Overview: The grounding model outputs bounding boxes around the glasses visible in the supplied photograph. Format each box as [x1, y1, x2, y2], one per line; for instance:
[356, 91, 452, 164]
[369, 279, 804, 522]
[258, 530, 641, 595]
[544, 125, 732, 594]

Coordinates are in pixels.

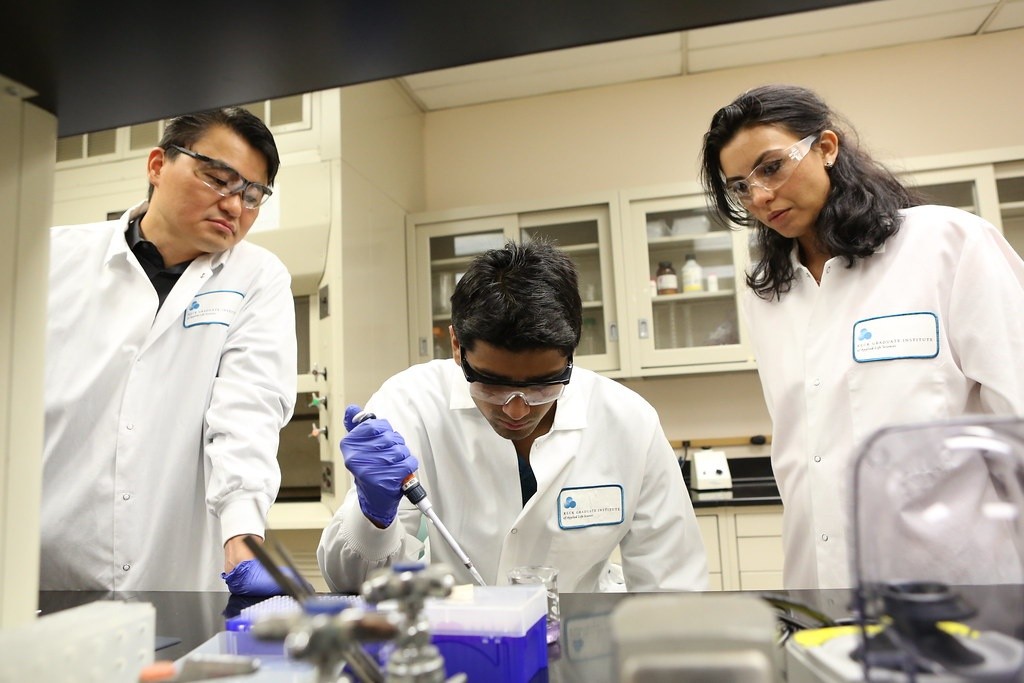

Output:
[726, 131, 821, 210]
[451, 324, 573, 407]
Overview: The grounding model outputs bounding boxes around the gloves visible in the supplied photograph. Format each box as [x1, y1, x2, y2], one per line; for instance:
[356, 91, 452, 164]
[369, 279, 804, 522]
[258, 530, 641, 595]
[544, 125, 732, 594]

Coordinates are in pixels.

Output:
[220, 555, 316, 598]
[164, 142, 273, 210]
[339, 404, 419, 527]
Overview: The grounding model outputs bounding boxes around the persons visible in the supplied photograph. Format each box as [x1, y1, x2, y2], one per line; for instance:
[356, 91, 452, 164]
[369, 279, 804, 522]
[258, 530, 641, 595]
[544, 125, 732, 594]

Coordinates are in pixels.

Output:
[316, 239, 712, 594]
[38, 107, 315, 595]
[700, 84, 1024, 587]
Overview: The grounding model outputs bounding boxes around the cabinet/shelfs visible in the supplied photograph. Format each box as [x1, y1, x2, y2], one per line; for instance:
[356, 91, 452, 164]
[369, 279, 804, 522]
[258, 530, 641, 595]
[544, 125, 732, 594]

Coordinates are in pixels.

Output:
[406, 190, 631, 379]
[620, 148, 1023, 377]
[690, 504, 786, 591]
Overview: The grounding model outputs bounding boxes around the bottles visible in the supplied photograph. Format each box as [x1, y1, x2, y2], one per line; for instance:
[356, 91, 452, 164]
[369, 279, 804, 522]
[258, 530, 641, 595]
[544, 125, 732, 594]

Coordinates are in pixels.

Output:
[681, 254, 704, 294]
[657, 261, 678, 295]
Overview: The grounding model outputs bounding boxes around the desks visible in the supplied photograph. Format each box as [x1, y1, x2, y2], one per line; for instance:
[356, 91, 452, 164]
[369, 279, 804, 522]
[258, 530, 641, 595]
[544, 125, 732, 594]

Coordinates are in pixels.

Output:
[36, 585, 1024, 683]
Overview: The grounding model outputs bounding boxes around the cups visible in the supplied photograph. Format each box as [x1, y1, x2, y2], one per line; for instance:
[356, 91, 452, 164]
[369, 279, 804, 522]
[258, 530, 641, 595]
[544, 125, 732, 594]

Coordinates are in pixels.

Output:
[506, 567, 561, 645]
